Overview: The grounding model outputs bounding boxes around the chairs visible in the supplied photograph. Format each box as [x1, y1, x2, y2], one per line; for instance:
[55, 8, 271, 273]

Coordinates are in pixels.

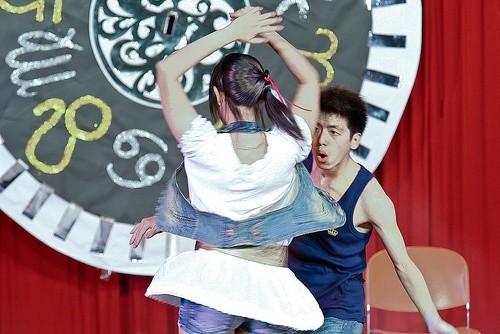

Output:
[366, 246, 481, 334]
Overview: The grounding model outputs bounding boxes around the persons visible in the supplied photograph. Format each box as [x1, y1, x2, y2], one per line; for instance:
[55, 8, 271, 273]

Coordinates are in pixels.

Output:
[144, 8, 346, 334]
[128, 87, 463, 334]
